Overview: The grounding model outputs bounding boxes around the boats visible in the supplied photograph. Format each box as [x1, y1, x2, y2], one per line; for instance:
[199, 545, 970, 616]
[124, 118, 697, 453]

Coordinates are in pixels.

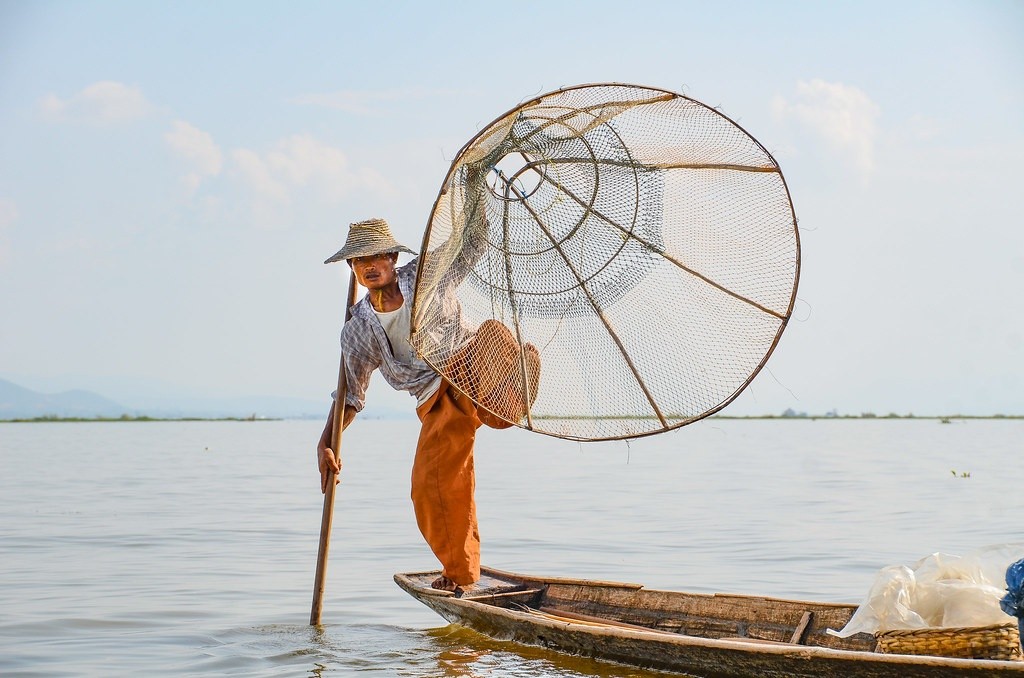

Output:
[391, 563, 1024, 678]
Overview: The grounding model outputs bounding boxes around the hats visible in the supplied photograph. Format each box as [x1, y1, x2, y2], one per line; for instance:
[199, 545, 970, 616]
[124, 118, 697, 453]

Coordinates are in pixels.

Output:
[324, 219, 419, 264]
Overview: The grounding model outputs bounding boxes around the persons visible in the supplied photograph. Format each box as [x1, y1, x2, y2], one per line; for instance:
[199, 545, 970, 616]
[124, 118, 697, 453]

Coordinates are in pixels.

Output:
[318, 161, 539, 589]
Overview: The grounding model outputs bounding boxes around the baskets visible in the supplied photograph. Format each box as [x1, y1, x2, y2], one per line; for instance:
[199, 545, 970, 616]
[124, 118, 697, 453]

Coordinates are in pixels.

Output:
[874, 622, 1022, 663]
